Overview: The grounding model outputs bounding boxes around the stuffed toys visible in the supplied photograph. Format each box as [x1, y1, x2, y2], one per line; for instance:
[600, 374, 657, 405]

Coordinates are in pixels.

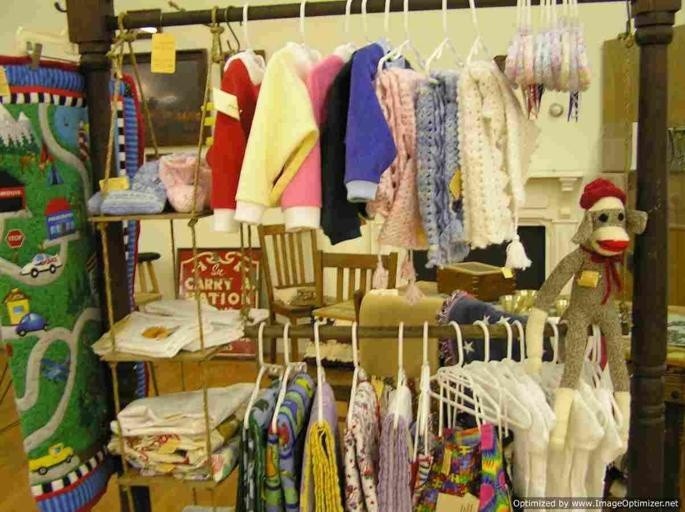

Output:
[524, 178, 648, 450]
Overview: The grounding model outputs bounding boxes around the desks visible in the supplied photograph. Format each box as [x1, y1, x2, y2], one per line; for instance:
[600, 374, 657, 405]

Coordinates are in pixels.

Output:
[311, 280, 684, 510]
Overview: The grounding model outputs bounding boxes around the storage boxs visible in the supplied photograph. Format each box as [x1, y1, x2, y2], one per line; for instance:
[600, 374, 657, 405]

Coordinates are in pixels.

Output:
[436, 260, 516, 302]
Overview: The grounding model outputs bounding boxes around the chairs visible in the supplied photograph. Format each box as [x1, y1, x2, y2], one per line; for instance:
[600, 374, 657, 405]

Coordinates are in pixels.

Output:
[302, 250, 398, 421]
[136, 251, 162, 305]
[257, 223, 336, 364]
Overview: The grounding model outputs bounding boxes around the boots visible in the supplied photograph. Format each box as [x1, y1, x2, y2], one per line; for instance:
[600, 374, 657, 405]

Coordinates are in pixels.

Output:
[159, 153, 204, 214]
